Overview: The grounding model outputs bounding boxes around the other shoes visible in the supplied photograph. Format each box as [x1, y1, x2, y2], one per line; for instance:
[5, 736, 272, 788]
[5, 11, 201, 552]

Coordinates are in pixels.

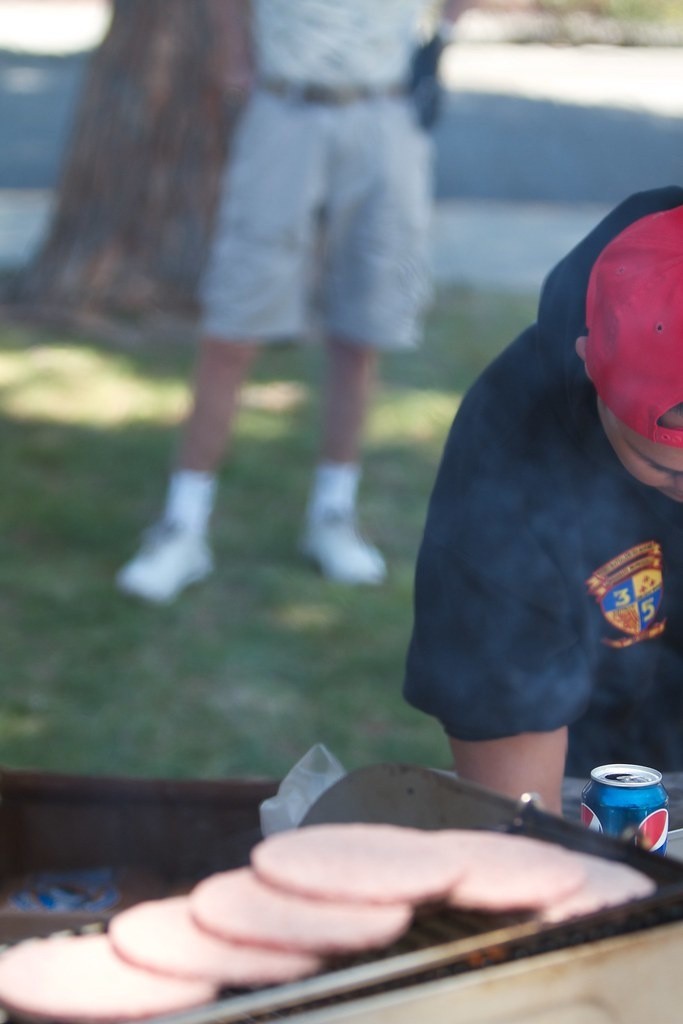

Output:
[114, 518, 214, 604]
[297, 508, 385, 585]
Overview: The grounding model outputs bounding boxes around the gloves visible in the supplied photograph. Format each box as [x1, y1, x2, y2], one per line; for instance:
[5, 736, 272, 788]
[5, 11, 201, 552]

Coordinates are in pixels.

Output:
[411, 39, 443, 128]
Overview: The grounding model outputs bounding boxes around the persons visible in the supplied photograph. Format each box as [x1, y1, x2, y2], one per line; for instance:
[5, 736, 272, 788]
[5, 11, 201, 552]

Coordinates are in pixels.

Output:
[403, 182, 682, 823]
[116, 0, 478, 610]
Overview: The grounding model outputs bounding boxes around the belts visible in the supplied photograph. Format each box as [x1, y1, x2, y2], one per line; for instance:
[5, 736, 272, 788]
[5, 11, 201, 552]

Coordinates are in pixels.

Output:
[255, 78, 411, 107]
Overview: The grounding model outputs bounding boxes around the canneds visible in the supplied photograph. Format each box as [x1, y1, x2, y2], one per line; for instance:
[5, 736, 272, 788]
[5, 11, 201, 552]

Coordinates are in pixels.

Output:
[580, 764, 669, 858]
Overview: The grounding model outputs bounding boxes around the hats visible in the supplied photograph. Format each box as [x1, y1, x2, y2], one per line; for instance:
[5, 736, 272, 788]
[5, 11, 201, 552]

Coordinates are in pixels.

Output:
[586, 204, 683, 448]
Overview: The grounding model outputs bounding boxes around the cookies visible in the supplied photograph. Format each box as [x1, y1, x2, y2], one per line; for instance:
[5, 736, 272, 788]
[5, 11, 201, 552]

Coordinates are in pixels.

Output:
[1, 820, 657, 1024]
